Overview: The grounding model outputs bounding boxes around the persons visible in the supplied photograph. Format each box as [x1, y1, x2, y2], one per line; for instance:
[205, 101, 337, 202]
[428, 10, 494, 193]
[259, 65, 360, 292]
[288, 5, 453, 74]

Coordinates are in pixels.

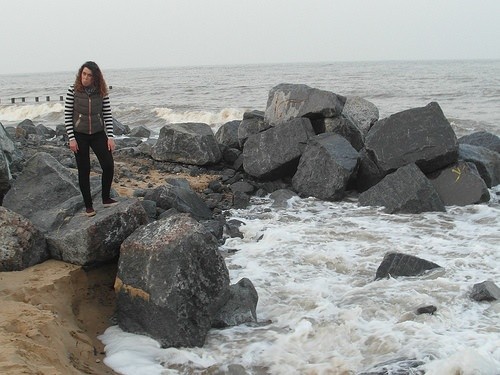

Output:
[63, 60, 121, 218]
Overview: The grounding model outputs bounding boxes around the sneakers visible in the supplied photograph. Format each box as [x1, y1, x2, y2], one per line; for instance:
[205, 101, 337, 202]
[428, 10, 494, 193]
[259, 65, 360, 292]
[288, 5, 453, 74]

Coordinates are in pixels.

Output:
[101, 198, 119, 208]
[84, 207, 96, 216]
